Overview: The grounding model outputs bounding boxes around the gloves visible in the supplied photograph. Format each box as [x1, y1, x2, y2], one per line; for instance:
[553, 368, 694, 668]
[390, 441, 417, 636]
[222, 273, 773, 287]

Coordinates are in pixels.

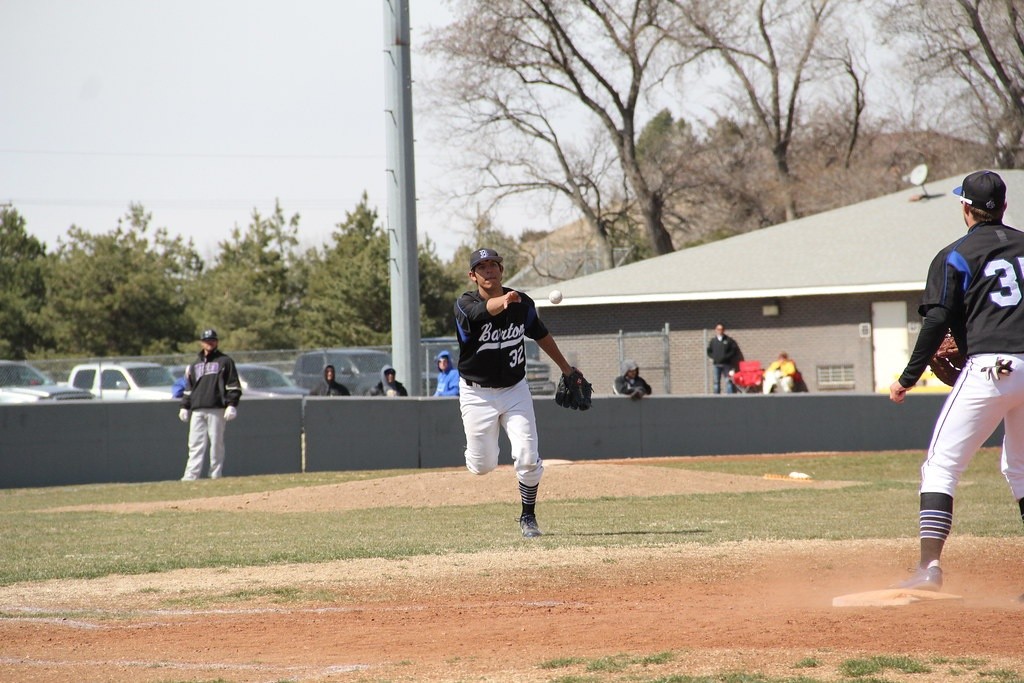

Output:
[178, 408, 189, 422]
[980, 357, 1014, 381]
[223, 406, 237, 421]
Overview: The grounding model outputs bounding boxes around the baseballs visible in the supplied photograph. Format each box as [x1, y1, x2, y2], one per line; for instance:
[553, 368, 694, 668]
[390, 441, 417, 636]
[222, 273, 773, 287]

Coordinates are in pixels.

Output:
[549, 290, 562, 304]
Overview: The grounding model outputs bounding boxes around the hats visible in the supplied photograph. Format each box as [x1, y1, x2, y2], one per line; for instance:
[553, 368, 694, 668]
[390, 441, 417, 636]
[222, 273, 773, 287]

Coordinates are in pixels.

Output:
[952, 169, 1006, 213]
[470, 248, 503, 270]
[201, 329, 218, 341]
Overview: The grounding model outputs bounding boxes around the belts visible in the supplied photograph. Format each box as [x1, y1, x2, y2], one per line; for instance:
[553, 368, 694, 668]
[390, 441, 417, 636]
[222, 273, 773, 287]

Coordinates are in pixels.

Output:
[465, 379, 501, 390]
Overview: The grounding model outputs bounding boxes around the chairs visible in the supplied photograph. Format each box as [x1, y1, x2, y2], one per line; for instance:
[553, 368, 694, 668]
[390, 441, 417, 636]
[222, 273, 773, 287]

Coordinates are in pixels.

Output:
[733, 362, 764, 397]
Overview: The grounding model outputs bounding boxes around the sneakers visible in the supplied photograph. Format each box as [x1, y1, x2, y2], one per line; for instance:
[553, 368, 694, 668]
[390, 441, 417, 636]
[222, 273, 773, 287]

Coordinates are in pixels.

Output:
[894, 564, 944, 591]
[515, 514, 542, 537]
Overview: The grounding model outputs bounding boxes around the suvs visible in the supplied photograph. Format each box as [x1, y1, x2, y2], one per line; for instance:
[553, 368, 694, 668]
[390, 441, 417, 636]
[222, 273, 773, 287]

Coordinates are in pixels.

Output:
[0, 361, 94, 403]
[168, 349, 556, 398]
[66, 361, 192, 400]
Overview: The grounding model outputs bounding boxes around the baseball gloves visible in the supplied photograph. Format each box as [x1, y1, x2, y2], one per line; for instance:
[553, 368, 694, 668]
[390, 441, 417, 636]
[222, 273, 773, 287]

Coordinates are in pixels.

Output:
[928, 327, 965, 387]
[555, 367, 592, 411]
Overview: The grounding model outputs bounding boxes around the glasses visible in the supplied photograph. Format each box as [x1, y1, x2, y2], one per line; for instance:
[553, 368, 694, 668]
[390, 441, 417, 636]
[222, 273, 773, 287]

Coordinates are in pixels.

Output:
[384, 370, 395, 375]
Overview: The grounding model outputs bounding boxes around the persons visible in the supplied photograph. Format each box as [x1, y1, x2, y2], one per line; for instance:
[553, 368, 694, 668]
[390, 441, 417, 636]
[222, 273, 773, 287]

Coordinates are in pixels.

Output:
[454, 248, 593, 537]
[707, 324, 737, 394]
[890, 169, 1024, 590]
[763, 350, 796, 395]
[363, 363, 408, 396]
[178, 328, 243, 482]
[172, 364, 190, 398]
[308, 363, 351, 397]
[432, 349, 459, 397]
[613, 360, 652, 402]
